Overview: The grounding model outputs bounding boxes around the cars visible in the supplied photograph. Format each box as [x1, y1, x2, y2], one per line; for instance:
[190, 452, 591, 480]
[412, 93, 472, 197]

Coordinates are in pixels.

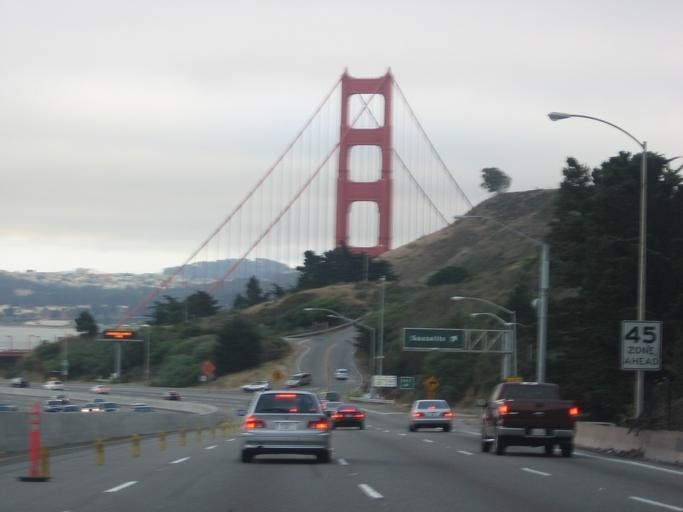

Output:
[238, 389, 365, 463]
[0, 377, 183, 414]
[408, 400, 455, 431]
[333, 367, 350, 382]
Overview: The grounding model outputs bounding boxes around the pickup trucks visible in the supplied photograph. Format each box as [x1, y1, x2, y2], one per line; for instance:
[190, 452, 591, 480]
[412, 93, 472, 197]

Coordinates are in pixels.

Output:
[480, 381, 578, 456]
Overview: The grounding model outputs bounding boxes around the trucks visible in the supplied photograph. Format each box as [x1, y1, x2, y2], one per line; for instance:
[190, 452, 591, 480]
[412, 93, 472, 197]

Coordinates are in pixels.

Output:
[240, 373, 313, 392]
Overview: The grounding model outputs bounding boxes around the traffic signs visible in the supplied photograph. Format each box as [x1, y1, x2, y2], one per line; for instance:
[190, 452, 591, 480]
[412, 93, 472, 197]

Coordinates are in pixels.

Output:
[422, 376, 440, 392]
[404, 326, 515, 355]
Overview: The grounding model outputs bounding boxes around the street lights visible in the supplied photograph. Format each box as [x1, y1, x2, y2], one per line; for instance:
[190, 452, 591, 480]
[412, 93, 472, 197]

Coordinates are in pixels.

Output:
[446, 211, 560, 384]
[549, 110, 655, 419]
[305, 275, 389, 376]
